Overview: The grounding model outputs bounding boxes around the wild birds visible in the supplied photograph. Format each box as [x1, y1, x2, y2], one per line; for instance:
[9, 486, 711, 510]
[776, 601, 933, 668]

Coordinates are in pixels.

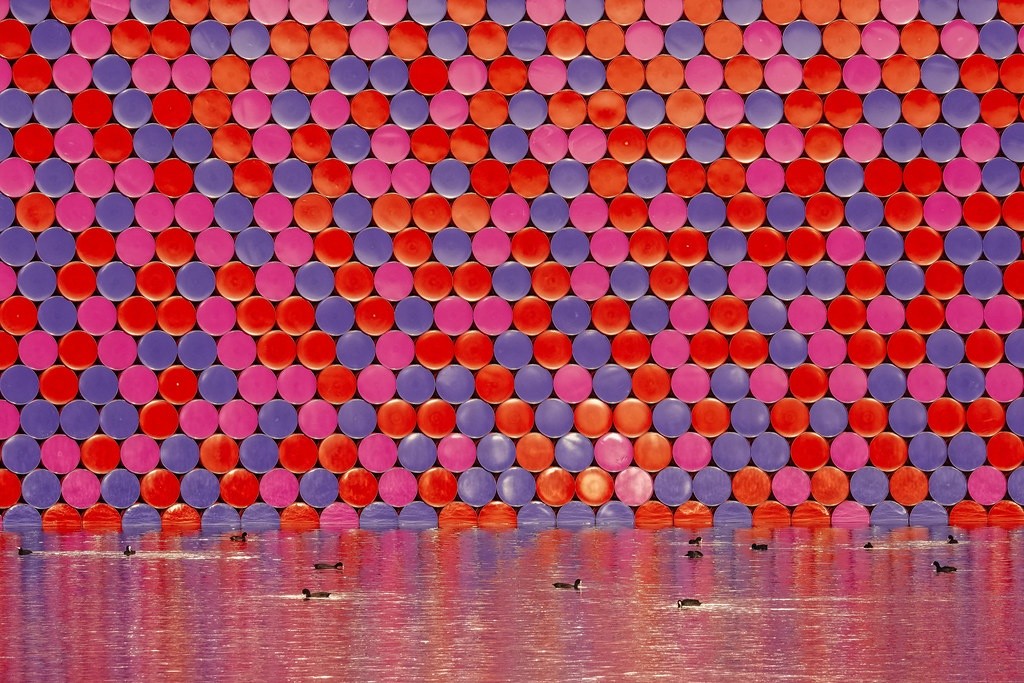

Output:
[124, 545, 136, 556]
[17, 546, 33, 555]
[930, 561, 957, 572]
[312, 562, 345, 569]
[947, 535, 959, 544]
[688, 537, 702, 544]
[230, 532, 248, 541]
[678, 598, 703, 608]
[684, 550, 704, 559]
[302, 589, 331, 598]
[551, 578, 582, 589]
[864, 542, 873, 548]
[752, 543, 768, 551]
